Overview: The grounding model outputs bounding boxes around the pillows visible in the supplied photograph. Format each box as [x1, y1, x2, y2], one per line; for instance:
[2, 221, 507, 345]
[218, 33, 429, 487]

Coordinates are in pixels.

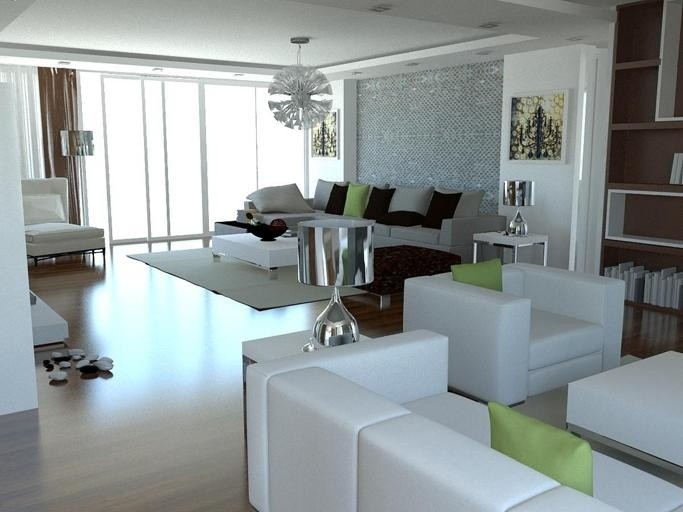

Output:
[451, 258, 503, 291]
[486, 400, 593, 498]
[245, 179, 483, 229]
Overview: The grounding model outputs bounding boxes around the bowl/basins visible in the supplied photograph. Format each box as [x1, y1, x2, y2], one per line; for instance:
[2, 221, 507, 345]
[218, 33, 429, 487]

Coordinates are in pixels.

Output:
[246, 223, 287, 240]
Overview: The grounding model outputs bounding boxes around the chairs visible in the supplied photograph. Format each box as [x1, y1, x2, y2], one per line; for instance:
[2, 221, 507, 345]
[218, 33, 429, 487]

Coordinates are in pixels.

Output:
[402, 262, 624, 406]
[20, 177, 107, 270]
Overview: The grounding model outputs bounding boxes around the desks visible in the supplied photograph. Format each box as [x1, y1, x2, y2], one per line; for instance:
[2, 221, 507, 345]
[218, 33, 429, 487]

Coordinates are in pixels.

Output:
[471, 231, 549, 267]
[242, 329, 373, 388]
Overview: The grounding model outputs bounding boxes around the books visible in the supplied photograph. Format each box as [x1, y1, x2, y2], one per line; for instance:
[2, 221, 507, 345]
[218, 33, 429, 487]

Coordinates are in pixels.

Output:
[668, 152, 683, 184]
[621, 306, 680, 350]
[601, 258, 683, 310]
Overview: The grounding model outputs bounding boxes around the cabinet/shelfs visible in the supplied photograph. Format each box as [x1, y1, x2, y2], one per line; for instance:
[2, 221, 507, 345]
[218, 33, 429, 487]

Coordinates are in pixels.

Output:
[602, 0, 681, 315]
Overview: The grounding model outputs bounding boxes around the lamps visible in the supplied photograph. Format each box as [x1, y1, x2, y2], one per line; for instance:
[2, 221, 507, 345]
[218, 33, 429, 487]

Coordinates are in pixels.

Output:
[503, 178, 535, 237]
[59, 128, 94, 225]
[266, 38, 332, 132]
[295, 221, 376, 351]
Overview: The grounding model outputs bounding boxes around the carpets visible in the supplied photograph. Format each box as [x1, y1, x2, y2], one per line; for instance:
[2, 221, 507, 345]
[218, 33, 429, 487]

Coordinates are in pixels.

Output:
[125, 247, 367, 311]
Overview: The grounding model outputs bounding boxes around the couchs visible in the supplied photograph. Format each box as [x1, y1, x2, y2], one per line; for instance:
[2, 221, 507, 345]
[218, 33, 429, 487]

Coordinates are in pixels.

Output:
[244, 328, 681, 512]
[215, 198, 498, 265]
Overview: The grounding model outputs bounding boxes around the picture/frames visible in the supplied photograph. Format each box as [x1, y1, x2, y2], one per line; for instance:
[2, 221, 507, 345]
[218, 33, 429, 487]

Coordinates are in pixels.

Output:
[309, 109, 339, 162]
[505, 89, 569, 163]
